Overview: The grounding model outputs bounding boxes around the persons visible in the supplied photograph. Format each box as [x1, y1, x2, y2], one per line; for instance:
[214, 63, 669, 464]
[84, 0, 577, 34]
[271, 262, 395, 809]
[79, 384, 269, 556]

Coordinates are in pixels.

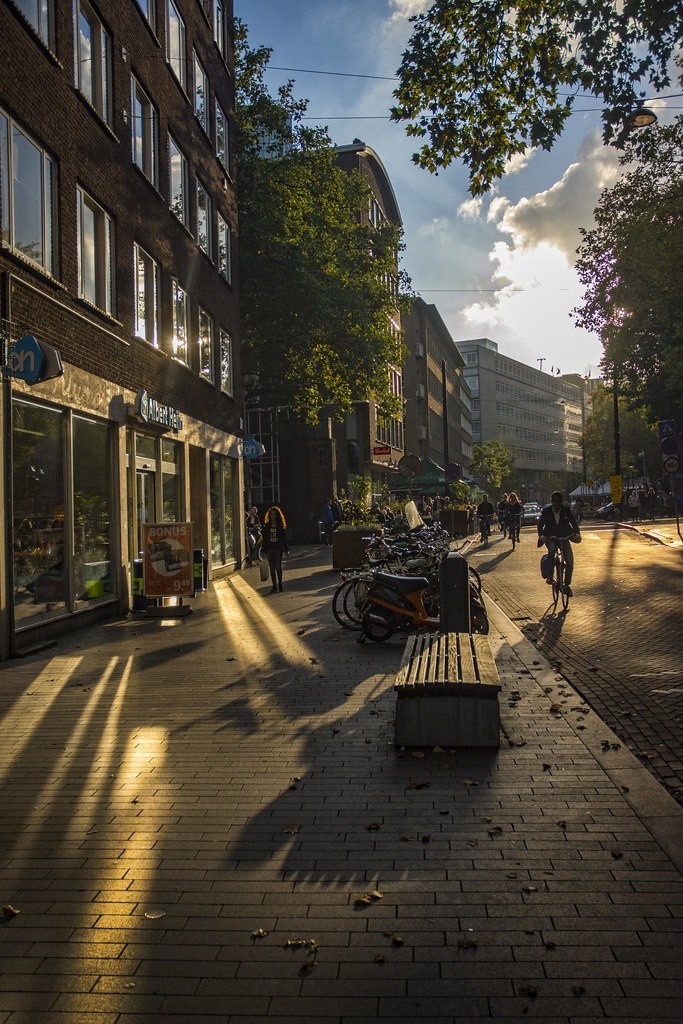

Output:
[262, 506, 290, 593]
[14, 519, 64, 578]
[320, 493, 457, 548]
[477, 495, 494, 542]
[573, 497, 584, 522]
[244, 507, 261, 567]
[537, 491, 582, 597]
[628, 488, 675, 524]
[496, 494, 509, 532]
[506, 492, 522, 543]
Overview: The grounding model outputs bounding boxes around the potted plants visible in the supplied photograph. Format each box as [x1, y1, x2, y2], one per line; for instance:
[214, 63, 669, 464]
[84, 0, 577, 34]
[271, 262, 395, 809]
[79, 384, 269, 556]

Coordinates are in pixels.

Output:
[434, 503, 469, 539]
[332, 522, 383, 572]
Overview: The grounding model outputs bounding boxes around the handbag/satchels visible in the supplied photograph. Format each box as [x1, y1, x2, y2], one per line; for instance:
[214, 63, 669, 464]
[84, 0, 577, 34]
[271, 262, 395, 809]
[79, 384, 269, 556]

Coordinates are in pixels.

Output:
[260, 554, 269, 582]
[541, 554, 551, 579]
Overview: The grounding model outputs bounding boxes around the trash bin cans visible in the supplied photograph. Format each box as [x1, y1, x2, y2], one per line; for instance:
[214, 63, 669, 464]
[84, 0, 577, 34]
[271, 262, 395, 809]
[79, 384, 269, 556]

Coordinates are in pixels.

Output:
[439, 552, 472, 634]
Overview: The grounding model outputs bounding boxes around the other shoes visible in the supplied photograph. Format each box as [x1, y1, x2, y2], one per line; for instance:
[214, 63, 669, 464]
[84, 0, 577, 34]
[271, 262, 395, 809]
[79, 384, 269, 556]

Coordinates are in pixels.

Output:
[546, 577, 553, 584]
[278, 582, 283, 592]
[271, 587, 277, 594]
[560, 586, 572, 597]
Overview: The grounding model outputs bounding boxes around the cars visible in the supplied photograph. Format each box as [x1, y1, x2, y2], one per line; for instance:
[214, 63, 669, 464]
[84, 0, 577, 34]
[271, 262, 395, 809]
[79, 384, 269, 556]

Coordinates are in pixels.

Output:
[521, 502, 543, 526]
[597, 501, 639, 520]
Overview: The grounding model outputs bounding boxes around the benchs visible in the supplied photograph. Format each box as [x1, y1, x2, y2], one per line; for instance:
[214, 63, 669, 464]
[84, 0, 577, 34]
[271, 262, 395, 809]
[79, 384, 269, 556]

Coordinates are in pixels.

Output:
[393, 631, 504, 753]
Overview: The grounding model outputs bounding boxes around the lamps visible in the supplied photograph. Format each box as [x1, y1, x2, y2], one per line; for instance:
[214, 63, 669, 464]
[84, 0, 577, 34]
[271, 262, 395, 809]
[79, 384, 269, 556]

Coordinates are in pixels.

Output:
[626, 100, 657, 128]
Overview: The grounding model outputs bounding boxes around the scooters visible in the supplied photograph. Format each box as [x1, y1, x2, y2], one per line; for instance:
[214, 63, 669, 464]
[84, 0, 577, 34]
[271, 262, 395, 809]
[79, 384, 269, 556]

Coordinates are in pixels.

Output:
[360, 569, 489, 641]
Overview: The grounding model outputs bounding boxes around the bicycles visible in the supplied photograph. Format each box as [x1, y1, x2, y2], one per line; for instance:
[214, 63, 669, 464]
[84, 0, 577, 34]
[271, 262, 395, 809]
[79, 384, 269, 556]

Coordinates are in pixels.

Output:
[332, 521, 489, 642]
[245, 525, 270, 565]
[480, 512, 522, 551]
[545, 535, 576, 609]
